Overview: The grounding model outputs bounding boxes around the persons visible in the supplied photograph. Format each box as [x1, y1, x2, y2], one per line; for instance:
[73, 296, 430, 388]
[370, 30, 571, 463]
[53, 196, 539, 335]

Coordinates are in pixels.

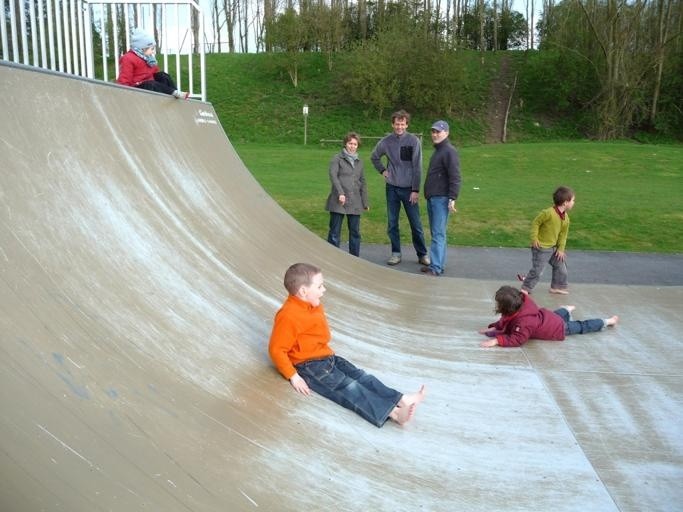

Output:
[424, 119, 462, 276]
[268, 263, 426, 429]
[322, 132, 370, 259]
[371, 109, 431, 268]
[114, 26, 191, 99]
[478, 286, 618, 346]
[521, 187, 577, 296]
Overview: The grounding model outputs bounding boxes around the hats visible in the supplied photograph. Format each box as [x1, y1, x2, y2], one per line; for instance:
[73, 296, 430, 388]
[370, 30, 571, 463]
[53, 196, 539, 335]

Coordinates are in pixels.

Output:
[431, 120, 449, 133]
[130, 27, 156, 50]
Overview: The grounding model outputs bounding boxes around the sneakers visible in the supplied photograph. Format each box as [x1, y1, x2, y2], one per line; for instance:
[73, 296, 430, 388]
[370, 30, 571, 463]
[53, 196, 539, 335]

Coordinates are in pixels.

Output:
[387, 255, 401, 265]
[418, 254, 430, 265]
